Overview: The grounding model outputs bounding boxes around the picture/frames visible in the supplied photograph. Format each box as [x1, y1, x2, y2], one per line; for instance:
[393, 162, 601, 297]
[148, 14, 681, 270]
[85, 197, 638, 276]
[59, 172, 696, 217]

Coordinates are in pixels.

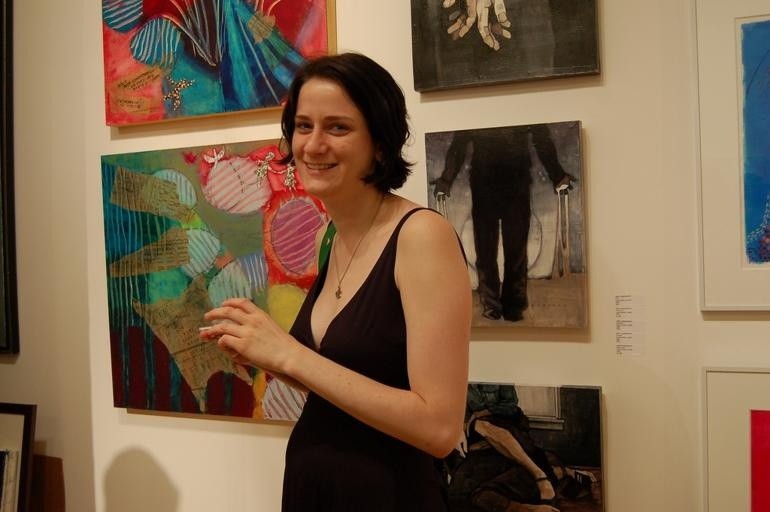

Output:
[693, 1, 770, 315]
[701, 366, 769, 512]
[0, 402, 38, 511]
[0, 0, 20, 357]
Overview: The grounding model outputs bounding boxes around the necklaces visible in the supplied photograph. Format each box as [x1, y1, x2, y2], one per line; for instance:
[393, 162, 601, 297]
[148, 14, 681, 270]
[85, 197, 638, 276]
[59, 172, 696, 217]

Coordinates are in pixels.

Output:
[333, 193, 386, 299]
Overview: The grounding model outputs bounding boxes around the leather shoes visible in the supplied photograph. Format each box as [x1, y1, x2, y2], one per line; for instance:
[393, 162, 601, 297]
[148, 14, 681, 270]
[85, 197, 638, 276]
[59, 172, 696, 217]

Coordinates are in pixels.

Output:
[481, 307, 523, 321]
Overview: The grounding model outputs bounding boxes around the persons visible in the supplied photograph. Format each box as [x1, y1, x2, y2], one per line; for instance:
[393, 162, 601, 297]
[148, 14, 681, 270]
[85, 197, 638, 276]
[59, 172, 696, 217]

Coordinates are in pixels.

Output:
[200, 54, 474, 512]
[429, 125, 577, 322]
[442, 0, 511, 51]
[455, 385, 558, 506]
